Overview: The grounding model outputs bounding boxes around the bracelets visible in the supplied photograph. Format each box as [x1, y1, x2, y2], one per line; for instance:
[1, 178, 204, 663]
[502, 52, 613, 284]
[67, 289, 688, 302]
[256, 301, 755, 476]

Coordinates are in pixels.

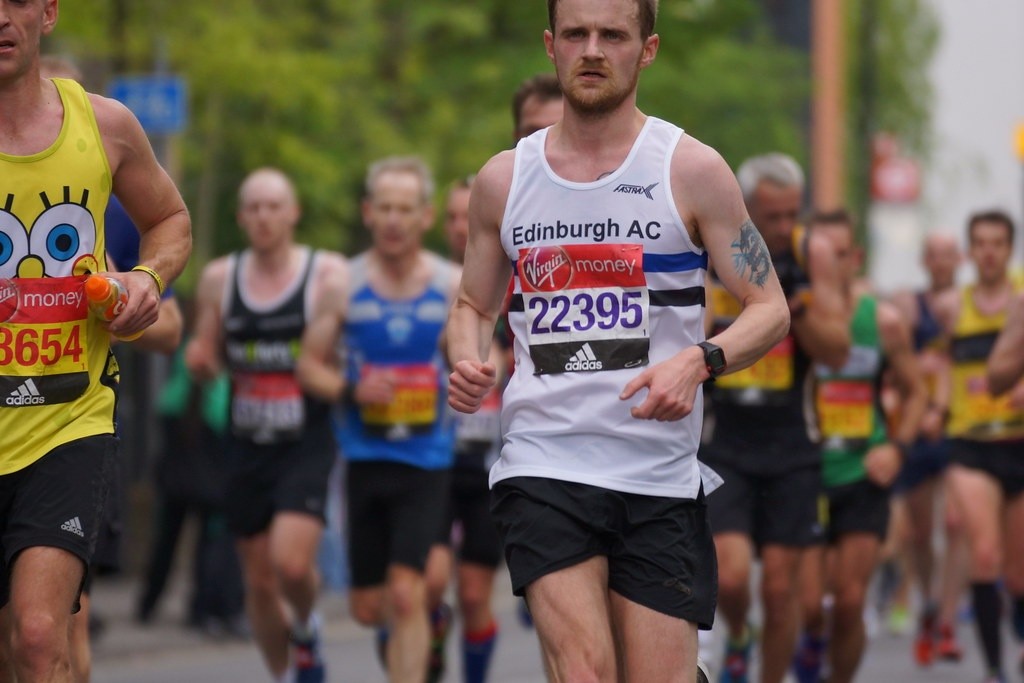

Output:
[341, 377, 357, 404]
[131, 266, 164, 296]
[892, 438, 908, 459]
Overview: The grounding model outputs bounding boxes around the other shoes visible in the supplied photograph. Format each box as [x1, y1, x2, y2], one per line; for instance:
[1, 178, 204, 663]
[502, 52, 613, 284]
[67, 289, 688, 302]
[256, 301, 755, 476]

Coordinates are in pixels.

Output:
[427, 602, 453, 683]
[792, 631, 828, 683]
[882, 604, 909, 637]
[717, 623, 754, 683]
[861, 604, 881, 641]
[694, 657, 711, 683]
[288, 613, 326, 683]
[375, 627, 389, 669]
[910, 620, 963, 669]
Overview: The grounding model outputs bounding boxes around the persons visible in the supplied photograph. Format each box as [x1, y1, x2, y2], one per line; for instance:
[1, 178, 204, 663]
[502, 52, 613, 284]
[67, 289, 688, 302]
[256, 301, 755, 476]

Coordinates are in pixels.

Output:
[293, 154, 462, 682]
[427, 311, 516, 683]
[186, 167, 348, 682]
[89, 197, 184, 636]
[445, 175, 516, 682]
[442, 0, 790, 683]
[512, 73, 564, 146]
[0, 0, 194, 682]
[698, 152, 1024, 683]
[133, 297, 252, 638]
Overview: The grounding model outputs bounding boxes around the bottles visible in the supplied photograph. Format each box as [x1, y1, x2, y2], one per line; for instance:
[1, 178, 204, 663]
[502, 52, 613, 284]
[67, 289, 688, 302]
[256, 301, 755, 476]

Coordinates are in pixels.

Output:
[85, 276, 147, 342]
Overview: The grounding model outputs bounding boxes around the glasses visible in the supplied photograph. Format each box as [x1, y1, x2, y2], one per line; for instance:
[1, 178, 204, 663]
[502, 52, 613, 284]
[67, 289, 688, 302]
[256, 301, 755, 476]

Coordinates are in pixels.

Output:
[836, 250, 850, 259]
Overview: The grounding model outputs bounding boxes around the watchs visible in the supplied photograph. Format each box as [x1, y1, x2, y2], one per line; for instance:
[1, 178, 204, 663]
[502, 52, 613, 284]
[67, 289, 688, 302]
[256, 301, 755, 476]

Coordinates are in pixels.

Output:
[698, 342, 727, 378]
